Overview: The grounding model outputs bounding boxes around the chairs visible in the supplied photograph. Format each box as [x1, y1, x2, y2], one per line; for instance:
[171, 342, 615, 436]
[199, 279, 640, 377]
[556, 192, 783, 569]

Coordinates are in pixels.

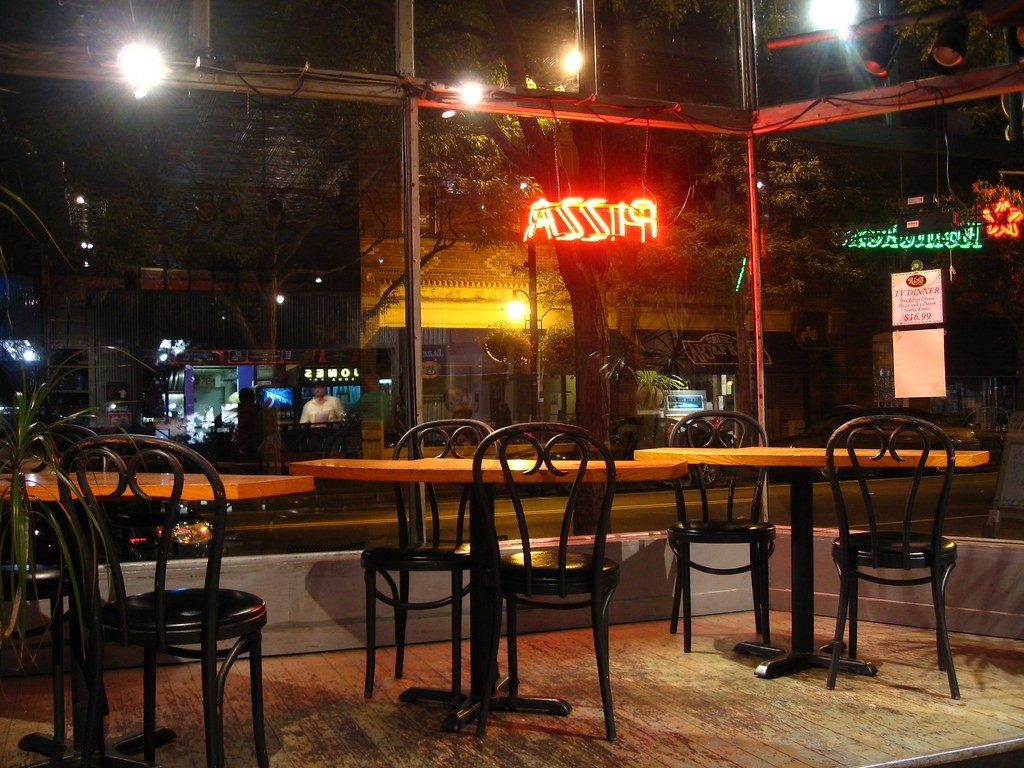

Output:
[0, 422, 111, 754]
[667, 410, 776, 652]
[359, 418, 501, 708]
[57, 433, 270, 768]
[826, 415, 962, 701]
[472, 421, 622, 740]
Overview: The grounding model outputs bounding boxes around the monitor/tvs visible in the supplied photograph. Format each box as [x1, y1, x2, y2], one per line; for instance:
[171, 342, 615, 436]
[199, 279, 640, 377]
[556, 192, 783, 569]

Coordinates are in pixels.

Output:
[256, 385, 299, 409]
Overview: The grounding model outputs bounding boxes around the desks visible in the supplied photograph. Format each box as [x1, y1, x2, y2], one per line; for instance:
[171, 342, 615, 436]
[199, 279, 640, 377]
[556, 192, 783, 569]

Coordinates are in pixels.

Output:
[0, 469, 316, 768]
[632, 448, 989, 680]
[288, 458, 690, 731]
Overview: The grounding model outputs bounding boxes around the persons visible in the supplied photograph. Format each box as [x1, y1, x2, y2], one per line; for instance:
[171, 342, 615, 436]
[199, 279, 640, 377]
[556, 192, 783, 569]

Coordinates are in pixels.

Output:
[299, 386, 346, 427]
